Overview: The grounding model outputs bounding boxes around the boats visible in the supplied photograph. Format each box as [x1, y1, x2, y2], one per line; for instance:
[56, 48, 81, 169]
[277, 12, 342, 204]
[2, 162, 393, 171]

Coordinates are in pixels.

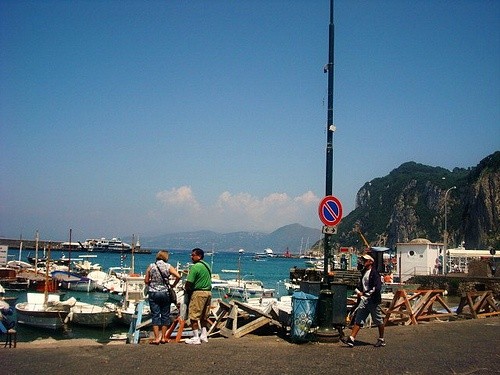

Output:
[184, 289, 421, 315]
[205, 250, 214, 255]
[69, 302, 117, 330]
[84, 237, 130, 249]
[115, 272, 178, 328]
[61, 240, 81, 245]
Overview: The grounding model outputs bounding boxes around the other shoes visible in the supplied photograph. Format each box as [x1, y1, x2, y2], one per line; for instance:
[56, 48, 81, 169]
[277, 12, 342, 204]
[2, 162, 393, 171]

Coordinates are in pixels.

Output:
[185, 337, 201, 345]
[199, 334, 209, 343]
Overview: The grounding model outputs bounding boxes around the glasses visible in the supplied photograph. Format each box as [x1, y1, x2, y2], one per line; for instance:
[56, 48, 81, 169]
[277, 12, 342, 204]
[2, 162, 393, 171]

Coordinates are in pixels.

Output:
[192, 254, 198, 257]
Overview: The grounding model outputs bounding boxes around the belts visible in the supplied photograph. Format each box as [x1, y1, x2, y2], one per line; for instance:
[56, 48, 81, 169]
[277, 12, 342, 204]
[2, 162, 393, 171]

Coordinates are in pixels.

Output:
[193, 288, 211, 291]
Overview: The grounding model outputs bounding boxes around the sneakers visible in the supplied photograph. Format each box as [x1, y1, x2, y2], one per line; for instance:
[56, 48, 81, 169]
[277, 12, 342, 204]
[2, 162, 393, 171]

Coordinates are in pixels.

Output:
[340, 336, 354, 347]
[374, 338, 386, 347]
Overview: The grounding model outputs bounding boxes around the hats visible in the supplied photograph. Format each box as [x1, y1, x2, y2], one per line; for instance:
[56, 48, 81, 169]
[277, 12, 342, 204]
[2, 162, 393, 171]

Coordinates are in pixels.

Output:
[361, 254, 374, 262]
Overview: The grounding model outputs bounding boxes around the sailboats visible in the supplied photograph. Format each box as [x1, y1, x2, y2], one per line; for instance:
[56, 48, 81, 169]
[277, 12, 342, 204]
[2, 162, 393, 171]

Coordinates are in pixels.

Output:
[8, 226, 275, 296]
[14, 244, 70, 332]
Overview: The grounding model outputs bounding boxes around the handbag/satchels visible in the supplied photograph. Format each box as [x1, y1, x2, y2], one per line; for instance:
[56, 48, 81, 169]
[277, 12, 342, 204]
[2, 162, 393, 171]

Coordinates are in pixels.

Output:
[168, 286, 177, 303]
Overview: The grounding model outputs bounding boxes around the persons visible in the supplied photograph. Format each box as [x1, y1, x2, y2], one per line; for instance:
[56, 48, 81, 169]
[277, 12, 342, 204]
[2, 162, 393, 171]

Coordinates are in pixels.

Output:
[185, 248, 212, 345]
[145, 250, 181, 343]
[339, 252, 386, 348]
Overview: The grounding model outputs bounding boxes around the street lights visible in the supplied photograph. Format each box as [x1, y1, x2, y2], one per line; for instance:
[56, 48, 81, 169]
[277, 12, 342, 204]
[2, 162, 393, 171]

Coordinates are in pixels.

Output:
[443, 186, 457, 273]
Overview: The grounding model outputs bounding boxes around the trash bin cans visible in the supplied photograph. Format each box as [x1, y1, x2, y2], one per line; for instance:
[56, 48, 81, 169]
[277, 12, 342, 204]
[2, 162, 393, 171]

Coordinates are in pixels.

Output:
[329, 283, 348, 324]
[291, 292, 318, 342]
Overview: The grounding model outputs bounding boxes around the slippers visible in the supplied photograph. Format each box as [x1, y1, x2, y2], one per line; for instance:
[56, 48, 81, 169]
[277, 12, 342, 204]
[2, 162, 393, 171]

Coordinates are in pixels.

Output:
[160, 339, 165, 344]
[149, 340, 159, 345]
